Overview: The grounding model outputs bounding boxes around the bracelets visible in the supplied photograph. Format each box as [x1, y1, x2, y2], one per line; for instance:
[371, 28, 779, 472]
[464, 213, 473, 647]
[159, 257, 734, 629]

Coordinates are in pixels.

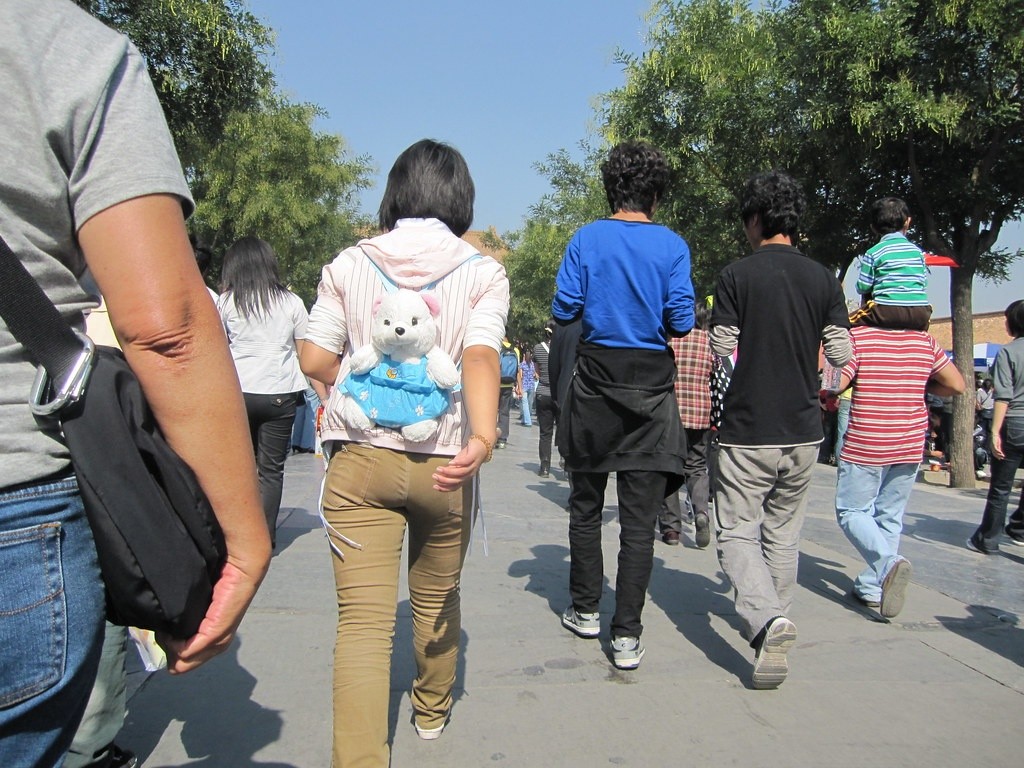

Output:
[991, 434, 1000, 437]
[464, 435, 493, 463]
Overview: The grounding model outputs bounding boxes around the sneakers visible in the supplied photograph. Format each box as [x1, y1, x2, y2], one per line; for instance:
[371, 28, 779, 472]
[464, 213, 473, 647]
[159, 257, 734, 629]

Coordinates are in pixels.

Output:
[966, 537, 998, 554]
[879, 561, 913, 619]
[562, 599, 601, 637]
[612, 637, 646, 669]
[752, 617, 799, 688]
[1005, 529, 1022, 545]
[852, 581, 878, 607]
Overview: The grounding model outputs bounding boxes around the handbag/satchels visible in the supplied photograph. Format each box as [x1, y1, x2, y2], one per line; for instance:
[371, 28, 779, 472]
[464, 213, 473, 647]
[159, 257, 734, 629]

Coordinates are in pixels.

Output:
[30, 336, 227, 642]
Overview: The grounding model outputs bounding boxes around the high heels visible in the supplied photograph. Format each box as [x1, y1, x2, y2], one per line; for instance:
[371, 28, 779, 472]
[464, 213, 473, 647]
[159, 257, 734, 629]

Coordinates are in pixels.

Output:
[292, 446, 313, 454]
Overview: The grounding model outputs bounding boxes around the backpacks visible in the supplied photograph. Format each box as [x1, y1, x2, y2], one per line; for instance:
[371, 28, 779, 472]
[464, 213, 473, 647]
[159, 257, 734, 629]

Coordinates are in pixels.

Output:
[350, 251, 483, 444]
[499, 342, 519, 383]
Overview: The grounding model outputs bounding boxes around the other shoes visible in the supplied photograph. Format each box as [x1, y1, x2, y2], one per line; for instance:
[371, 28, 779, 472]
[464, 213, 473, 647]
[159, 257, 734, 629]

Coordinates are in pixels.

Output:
[695, 512, 711, 548]
[412, 699, 446, 740]
[496, 442, 506, 451]
[662, 531, 682, 544]
[97, 745, 139, 766]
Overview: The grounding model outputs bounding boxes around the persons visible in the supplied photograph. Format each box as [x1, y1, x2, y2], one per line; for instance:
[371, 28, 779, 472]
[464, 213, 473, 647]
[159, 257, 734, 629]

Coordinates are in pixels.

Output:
[1, 1, 1023, 767]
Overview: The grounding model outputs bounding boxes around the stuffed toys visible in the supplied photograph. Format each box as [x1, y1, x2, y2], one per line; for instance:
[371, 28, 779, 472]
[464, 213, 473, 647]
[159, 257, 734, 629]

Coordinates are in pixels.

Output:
[337, 286, 460, 444]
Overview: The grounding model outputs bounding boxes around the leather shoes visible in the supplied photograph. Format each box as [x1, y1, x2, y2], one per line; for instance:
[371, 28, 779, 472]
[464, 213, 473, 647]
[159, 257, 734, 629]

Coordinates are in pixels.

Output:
[538, 469, 550, 478]
